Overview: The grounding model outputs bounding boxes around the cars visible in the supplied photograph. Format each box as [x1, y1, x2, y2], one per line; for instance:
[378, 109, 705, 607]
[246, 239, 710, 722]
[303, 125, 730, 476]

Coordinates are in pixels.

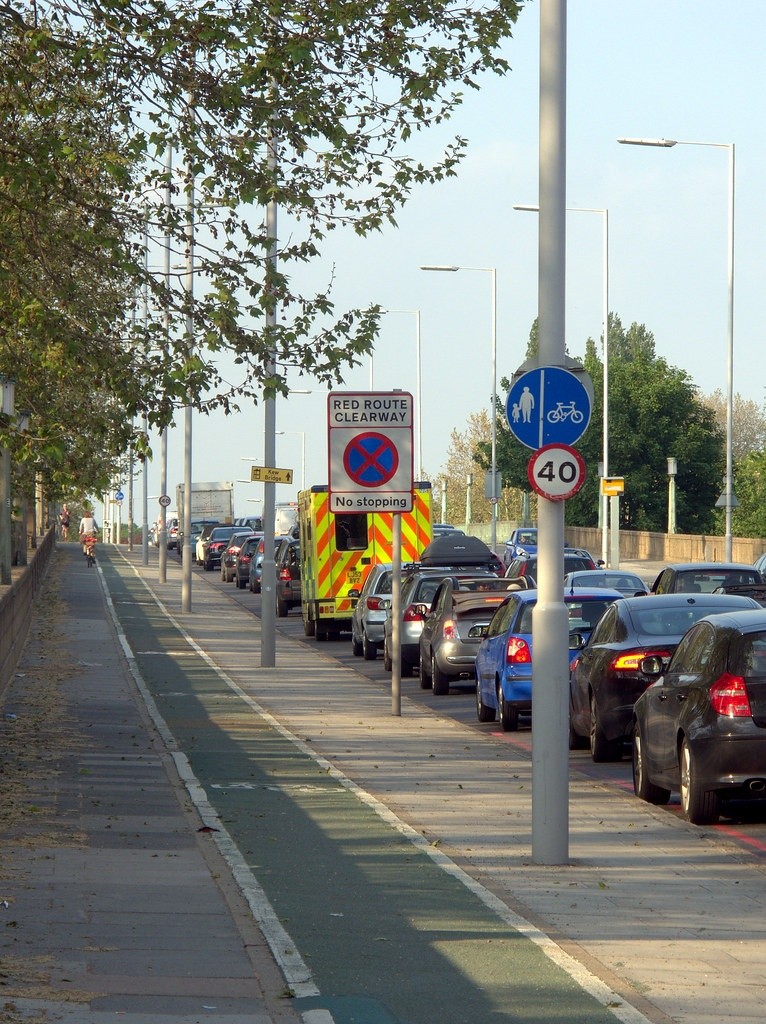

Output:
[564, 571, 652, 629]
[412, 577, 528, 694]
[505, 526, 568, 567]
[632, 564, 765, 608]
[468, 584, 626, 732]
[150, 517, 287, 593]
[712, 584, 766, 609]
[274, 534, 302, 617]
[747, 554, 766, 583]
[568, 592, 764, 764]
[348, 565, 450, 661]
[632, 610, 766, 824]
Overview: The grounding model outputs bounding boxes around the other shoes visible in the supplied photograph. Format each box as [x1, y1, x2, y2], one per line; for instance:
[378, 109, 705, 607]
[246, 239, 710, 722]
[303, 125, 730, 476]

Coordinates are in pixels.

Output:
[62, 536, 64, 541]
[84, 553, 87, 555]
[90, 556, 95, 563]
[65, 537, 67, 543]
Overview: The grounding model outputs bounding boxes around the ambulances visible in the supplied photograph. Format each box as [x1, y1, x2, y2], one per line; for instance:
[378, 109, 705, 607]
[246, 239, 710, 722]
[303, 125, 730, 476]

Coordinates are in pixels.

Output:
[292, 481, 432, 641]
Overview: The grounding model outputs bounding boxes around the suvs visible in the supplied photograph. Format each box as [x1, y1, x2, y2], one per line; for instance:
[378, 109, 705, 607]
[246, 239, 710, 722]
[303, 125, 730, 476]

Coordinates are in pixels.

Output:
[504, 549, 606, 587]
[377, 535, 501, 677]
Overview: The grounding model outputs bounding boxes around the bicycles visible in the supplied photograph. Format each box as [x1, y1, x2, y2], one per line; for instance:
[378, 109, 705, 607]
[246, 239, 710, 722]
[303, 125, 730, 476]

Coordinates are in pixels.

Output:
[87, 545, 94, 568]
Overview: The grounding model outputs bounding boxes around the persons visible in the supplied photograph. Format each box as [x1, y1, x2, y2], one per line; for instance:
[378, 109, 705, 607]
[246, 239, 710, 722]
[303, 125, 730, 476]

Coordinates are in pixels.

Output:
[519, 536, 536, 544]
[79, 511, 99, 564]
[59, 504, 71, 538]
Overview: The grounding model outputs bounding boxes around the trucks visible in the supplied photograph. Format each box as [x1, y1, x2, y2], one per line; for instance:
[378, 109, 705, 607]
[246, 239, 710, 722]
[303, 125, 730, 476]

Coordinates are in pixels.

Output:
[273, 502, 299, 536]
[176, 482, 234, 555]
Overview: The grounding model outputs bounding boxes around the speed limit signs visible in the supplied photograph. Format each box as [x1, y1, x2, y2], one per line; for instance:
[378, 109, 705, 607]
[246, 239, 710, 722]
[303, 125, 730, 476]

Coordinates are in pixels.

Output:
[527, 444, 585, 500]
[117, 500, 123, 506]
[159, 496, 171, 507]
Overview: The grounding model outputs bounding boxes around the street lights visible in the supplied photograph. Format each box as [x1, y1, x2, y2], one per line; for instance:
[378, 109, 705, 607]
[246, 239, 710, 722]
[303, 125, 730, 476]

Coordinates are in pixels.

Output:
[274, 430, 305, 492]
[511, 203, 608, 561]
[420, 264, 497, 551]
[247, 499, 263, 518]
[360, 309, 422, 484]
[620, 135, 735, 563]
